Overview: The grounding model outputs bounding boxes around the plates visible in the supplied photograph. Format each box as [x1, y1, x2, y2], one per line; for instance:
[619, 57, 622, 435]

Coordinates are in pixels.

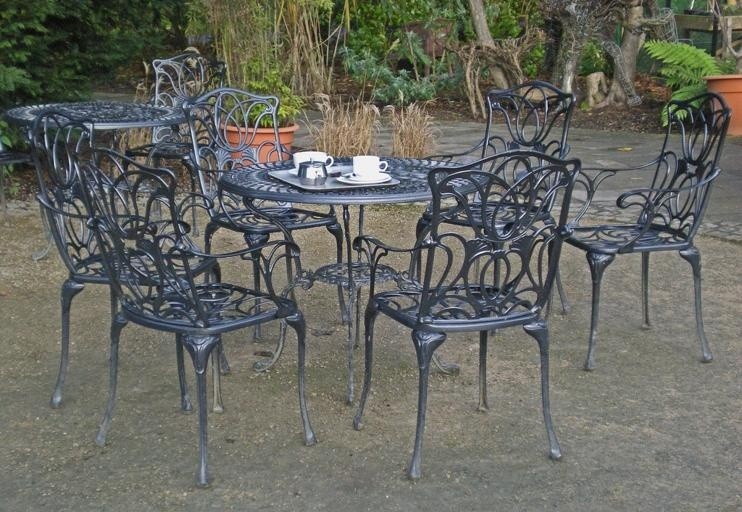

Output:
[288, 167, 339, 177]
[337, 172, 392, 185]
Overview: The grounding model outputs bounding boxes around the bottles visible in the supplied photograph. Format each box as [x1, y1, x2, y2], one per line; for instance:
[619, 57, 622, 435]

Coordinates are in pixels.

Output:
[298, 158, 328, 186]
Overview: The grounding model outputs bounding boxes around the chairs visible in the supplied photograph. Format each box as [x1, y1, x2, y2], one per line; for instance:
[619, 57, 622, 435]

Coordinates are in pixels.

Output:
[351, 148, 582, 481]
[546, 93, 732, 372]
[70, 146, 318, 486]
[182, 87, 352, 344]
[416, 79, 576, 317]
[8, 53, 228, 272]
[22, 106, 230, 413]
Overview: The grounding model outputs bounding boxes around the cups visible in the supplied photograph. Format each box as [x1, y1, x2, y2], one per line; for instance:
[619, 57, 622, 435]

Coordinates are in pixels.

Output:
[352, 155, 388, 179]
[292, 151, 335, 171]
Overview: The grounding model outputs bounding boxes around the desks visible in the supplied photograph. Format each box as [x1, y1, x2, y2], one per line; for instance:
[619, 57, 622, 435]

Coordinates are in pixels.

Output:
[217, 154, 491, 404]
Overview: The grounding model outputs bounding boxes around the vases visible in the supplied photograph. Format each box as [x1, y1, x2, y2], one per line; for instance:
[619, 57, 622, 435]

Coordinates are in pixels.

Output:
[704, 77, 742, 137]
